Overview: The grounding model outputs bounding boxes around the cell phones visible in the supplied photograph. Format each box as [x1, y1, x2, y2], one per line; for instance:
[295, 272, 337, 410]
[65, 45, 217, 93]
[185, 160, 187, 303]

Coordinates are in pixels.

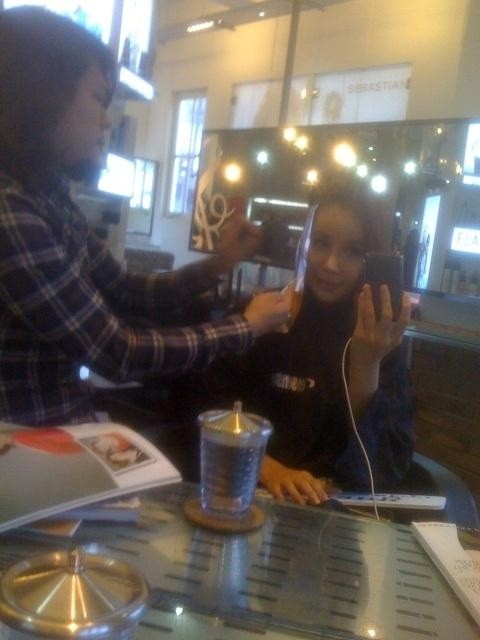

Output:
[365, 253, 403, 322]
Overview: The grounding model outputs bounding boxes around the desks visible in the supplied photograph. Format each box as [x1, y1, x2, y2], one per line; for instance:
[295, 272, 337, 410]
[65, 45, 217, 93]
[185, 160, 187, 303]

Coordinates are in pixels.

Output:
[0, 482, 480, 640]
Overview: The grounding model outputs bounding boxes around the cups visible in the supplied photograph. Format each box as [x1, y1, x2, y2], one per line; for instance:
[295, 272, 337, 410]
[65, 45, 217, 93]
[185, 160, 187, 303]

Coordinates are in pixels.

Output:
[196, 400, 274, 520]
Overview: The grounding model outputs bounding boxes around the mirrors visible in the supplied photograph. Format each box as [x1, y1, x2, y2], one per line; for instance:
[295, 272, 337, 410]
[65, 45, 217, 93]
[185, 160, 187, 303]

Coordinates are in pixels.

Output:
[187, 116, 479, 307]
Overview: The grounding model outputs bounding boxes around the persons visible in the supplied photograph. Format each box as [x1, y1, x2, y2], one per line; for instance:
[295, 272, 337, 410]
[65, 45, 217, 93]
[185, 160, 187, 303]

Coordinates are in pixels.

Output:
[0, 5, 294, 431]
[165, 175, 413, 524]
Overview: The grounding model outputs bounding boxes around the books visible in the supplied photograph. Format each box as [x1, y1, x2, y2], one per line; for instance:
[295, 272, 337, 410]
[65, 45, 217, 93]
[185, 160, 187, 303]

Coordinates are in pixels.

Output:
[4, 433, 188, 542]
